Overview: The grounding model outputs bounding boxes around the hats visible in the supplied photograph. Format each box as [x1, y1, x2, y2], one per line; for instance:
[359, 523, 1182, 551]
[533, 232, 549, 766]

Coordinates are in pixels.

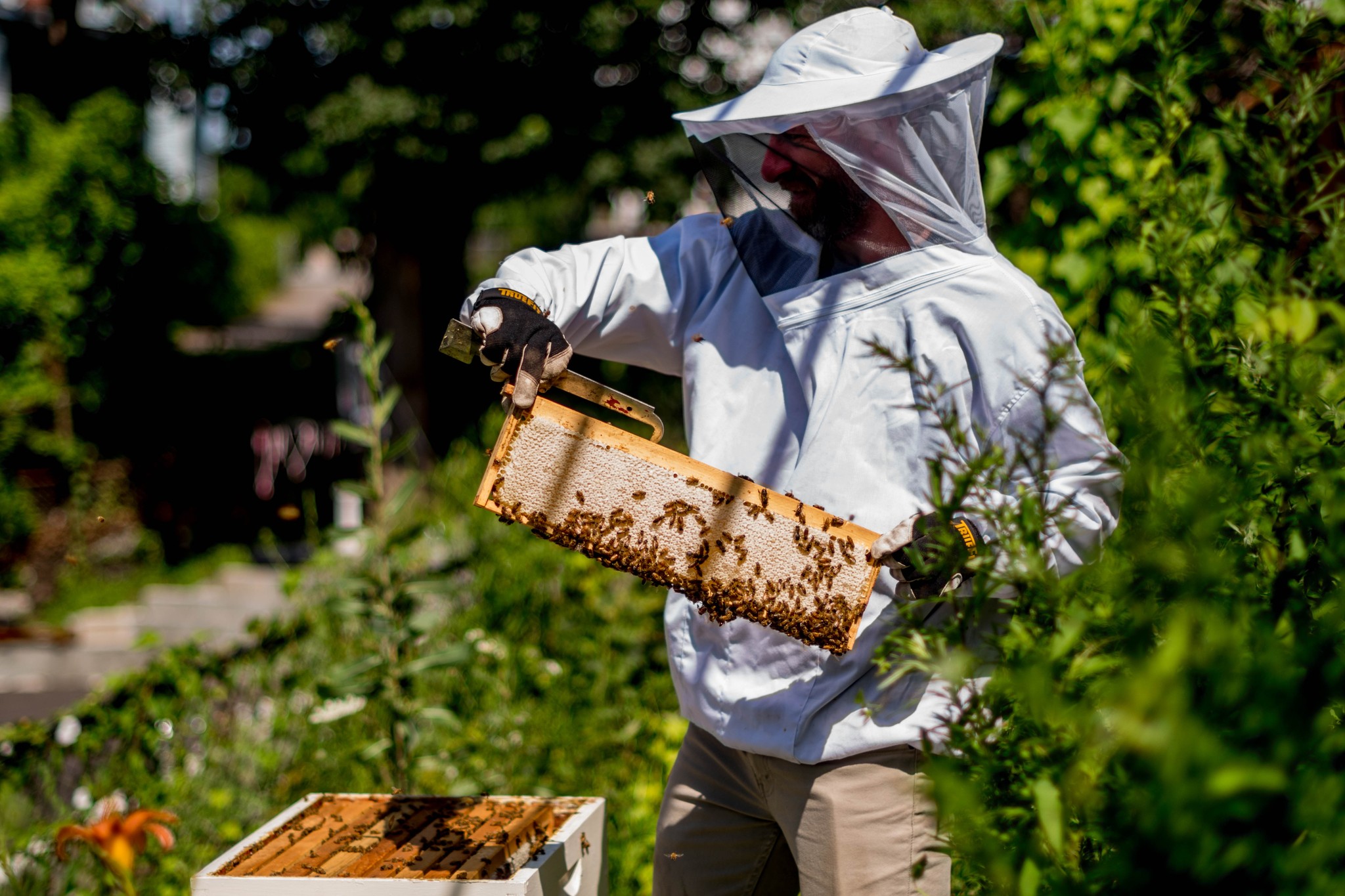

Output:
[670, 7, 1005, 121]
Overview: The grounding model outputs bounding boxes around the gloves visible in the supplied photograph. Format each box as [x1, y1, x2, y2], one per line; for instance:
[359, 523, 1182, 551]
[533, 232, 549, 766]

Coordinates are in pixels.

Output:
[867, 510, 1002, 628]
[468, 288, 573, 411]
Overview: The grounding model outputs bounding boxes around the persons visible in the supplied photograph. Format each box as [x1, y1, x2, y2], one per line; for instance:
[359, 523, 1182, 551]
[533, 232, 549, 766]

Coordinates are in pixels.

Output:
[458, 5, 1123, 895]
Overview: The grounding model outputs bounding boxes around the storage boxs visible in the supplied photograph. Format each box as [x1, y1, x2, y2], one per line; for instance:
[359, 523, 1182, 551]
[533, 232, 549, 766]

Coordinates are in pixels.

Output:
[190, 793, 607, 896]
